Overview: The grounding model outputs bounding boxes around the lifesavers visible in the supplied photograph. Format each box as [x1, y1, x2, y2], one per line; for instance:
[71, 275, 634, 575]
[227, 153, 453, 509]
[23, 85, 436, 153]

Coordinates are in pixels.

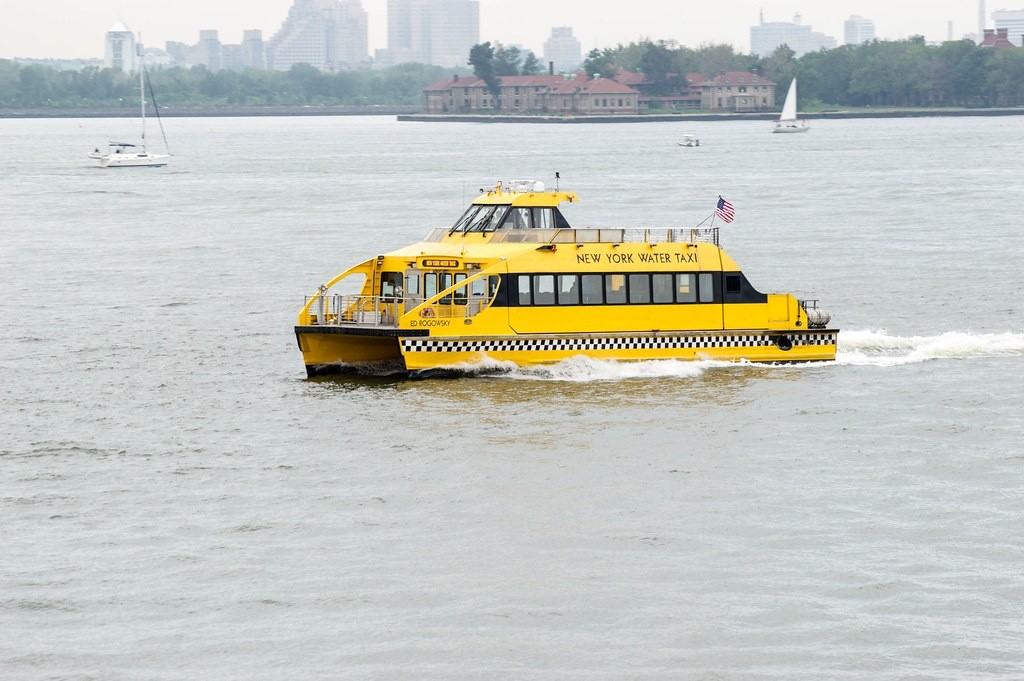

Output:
[421, 307, 435, 316]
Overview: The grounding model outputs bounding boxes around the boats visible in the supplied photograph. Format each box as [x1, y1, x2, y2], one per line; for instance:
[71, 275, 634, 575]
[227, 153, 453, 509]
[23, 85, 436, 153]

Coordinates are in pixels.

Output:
[678, 134, 699, 148]
[290, 176, 845, 371]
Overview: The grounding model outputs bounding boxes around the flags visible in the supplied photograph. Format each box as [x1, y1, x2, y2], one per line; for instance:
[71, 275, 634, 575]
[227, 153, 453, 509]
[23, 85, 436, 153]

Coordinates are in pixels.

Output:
[714, 195, 735, 223]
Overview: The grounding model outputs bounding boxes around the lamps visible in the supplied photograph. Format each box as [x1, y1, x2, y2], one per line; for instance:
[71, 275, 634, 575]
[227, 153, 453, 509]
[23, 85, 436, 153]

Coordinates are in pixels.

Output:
[378, 255, 384, 260]
[377, 261, 383, 265]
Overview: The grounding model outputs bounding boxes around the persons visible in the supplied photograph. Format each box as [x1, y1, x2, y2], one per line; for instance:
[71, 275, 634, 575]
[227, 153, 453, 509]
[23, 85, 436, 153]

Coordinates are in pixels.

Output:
[95, 147, 98, 152]
[394, 286, 402, 297]
[116, 149, 119, 153]
[570, 282, 576, 292]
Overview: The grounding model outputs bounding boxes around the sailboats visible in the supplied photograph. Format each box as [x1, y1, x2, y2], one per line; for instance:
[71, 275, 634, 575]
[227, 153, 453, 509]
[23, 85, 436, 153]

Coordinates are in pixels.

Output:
[768, 75, 811, 132]
[87, 32, 184, 168]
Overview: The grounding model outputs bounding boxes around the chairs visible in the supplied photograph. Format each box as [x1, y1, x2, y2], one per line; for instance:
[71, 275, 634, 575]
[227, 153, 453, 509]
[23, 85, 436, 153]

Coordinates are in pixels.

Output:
[446, 292, 665, 305]
